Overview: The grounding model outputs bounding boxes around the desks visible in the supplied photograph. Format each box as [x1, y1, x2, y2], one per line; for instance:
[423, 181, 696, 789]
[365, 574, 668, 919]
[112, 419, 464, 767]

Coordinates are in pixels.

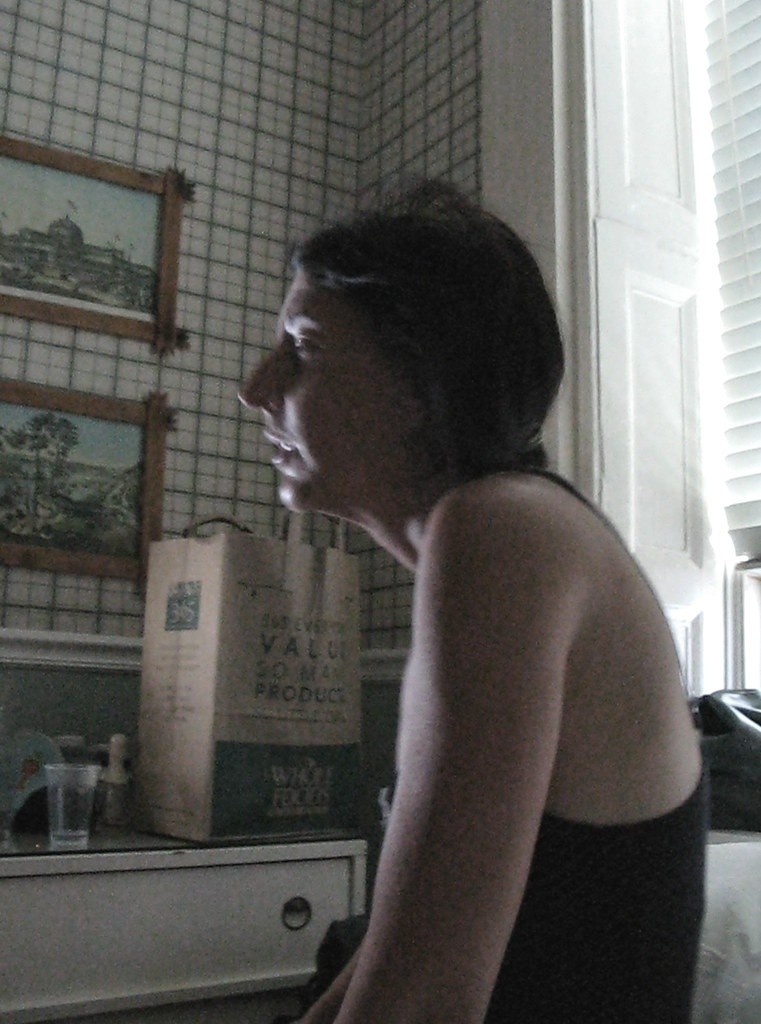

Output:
[0, 824, 367, 1024]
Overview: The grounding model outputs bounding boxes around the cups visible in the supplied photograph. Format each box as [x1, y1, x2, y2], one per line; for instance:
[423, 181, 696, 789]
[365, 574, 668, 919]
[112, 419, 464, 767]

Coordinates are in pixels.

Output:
[43, 764, 100, 851]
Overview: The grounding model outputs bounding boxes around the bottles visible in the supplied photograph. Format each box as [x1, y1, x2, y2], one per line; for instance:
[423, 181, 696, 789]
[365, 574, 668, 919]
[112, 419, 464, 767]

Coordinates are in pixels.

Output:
[102, 734, 129, 826]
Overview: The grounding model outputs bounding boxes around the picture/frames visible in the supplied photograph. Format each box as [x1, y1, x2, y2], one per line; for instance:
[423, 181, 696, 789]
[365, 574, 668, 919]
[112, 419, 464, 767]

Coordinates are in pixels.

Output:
[0, 134, 196, 359]
[0, 377, 177, 600]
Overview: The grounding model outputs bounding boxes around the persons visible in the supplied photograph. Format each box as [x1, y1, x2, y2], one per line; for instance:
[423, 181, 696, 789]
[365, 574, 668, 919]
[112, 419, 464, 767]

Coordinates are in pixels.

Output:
[236, 180, 708, 1024]
